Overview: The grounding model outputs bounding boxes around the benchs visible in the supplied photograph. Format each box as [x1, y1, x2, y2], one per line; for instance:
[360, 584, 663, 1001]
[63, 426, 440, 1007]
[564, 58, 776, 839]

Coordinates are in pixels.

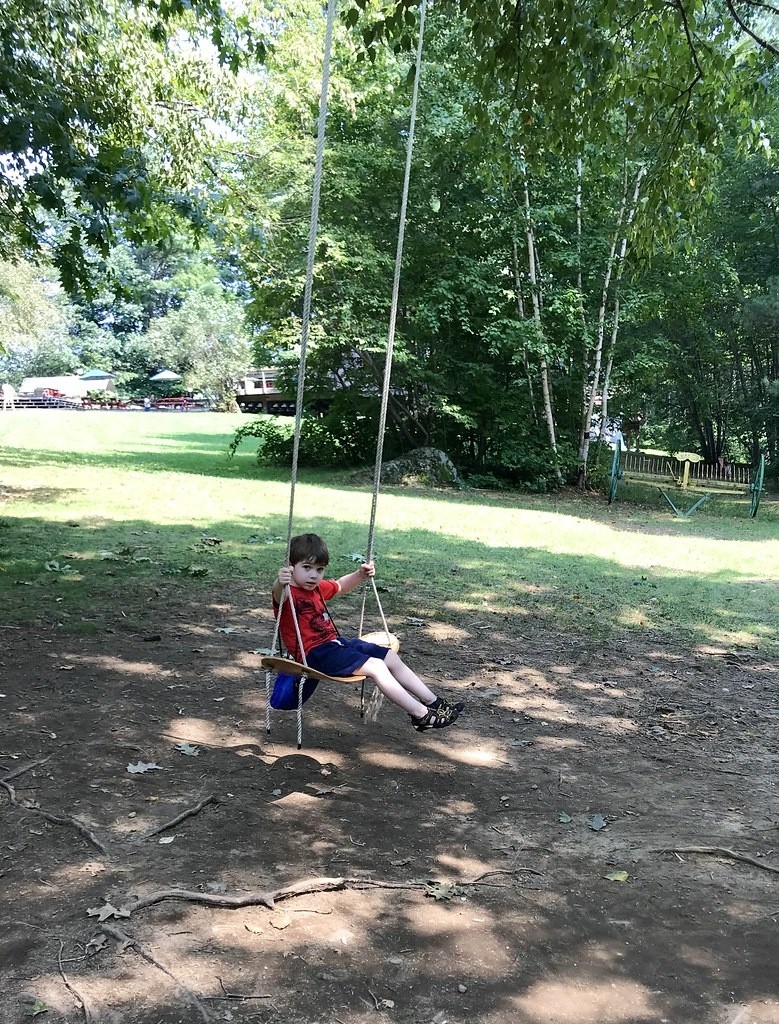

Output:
[607, 438, 765, 519]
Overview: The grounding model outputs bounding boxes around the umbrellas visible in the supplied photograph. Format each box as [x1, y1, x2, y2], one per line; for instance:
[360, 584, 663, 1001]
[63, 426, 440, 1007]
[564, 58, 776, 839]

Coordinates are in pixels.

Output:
[150, 370, 183, 398]
[78, 368, 117, 380]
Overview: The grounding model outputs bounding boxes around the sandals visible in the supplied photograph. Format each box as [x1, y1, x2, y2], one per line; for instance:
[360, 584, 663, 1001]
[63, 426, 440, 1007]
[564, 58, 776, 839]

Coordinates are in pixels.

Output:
[420, 696, 465, 713]
[407, 707, 459, 732]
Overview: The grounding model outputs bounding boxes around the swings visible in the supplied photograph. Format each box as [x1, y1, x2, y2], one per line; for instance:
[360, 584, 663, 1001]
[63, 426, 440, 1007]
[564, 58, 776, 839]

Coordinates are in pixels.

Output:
[260, 0, 434, 684]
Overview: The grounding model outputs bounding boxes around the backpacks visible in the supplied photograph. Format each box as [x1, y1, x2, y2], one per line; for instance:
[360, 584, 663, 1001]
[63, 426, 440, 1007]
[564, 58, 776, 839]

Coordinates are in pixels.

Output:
[270, 586, 340, 710]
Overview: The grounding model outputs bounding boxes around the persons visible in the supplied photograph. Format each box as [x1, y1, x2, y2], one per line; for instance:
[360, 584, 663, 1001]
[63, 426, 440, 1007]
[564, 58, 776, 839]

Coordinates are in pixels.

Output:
[2, 379, 17, 411]
[109, 390, 203, 410]
[272, 533, 465, 733]
[42, 389, 63, 398]
